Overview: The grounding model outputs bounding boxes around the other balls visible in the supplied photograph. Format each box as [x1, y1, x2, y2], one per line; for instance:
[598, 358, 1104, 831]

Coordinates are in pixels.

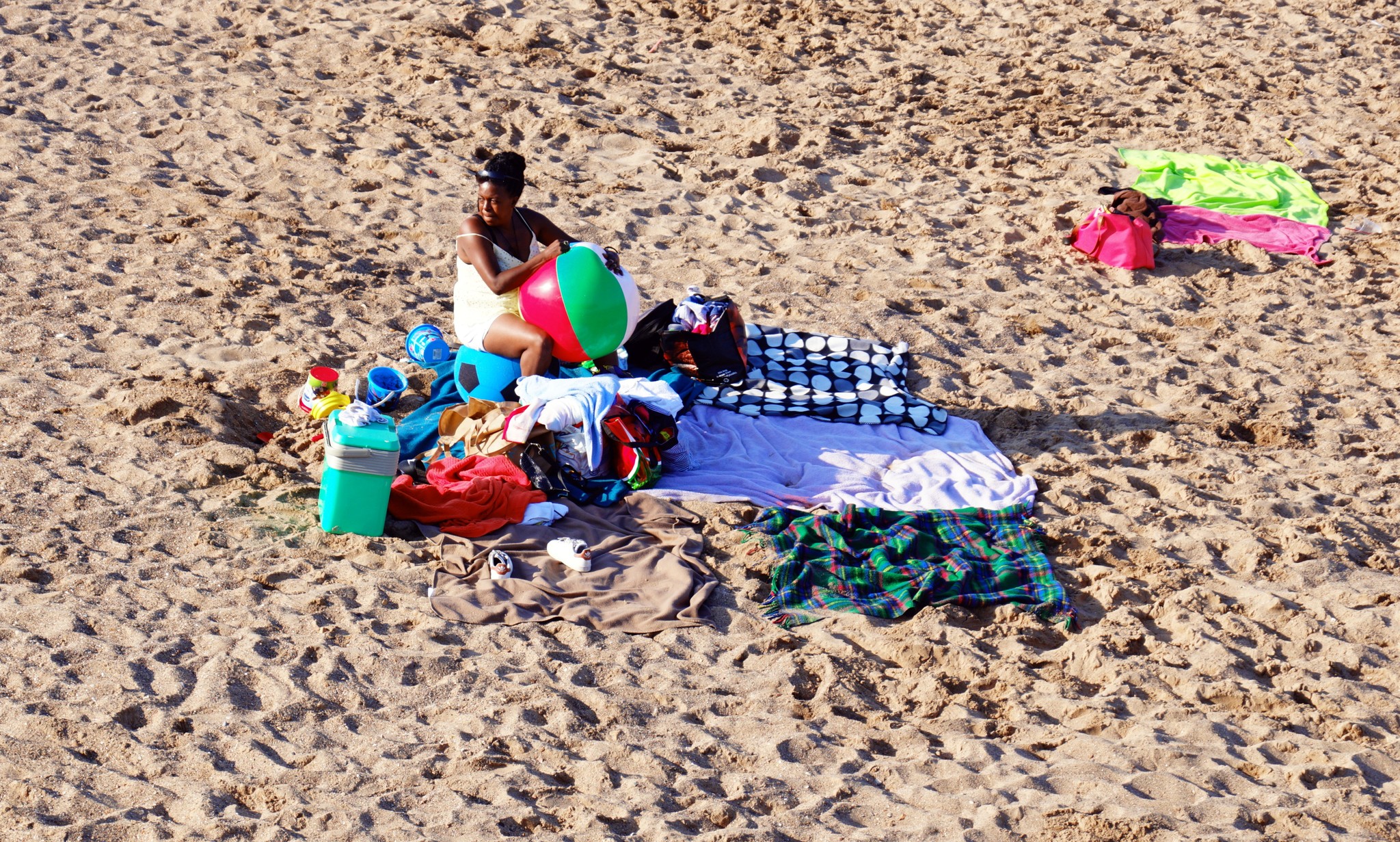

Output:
[517, 241, 640, 363]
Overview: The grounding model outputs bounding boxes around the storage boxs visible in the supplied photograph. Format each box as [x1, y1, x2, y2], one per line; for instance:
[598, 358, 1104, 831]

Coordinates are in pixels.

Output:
[319, 410, 401, 537]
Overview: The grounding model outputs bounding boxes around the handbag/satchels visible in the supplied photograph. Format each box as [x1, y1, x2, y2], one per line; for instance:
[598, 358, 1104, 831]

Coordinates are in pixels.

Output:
[601, 404, 662, 490]
[601, 397, 700, 472]
[660, 293, 747, 386]
[624, 298, 677, 372]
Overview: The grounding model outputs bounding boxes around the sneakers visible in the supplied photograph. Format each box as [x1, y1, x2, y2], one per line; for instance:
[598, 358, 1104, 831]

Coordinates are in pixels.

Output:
[488, 550, 513, 579]
[547, 537, 592, 572]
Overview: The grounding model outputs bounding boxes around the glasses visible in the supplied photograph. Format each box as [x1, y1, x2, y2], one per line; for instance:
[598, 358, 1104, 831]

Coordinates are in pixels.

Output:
[475, 170, 521, 186]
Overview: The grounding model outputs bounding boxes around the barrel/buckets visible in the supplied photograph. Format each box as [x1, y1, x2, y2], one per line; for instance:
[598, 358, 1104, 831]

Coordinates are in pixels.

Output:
[298, 366, 338, 413]
[405, 324, 454, 363]
[354, 367, 408, 414]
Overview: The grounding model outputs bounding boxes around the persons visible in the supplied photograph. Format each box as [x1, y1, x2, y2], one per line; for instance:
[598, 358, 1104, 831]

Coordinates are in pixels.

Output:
[452, 150, 624, 376]
